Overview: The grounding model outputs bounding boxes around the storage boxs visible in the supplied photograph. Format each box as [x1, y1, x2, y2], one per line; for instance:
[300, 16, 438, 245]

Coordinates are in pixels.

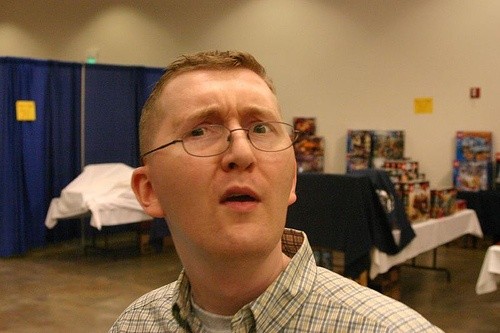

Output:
[344, 125, 493, 217]
[289, 117, 326, 175]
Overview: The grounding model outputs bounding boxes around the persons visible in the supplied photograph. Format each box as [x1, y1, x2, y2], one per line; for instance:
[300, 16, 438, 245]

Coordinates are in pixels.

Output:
[107, 50, 447, 332]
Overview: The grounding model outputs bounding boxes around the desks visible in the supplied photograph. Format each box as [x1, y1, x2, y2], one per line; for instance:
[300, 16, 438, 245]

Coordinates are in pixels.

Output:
[370, 209, 483, 289]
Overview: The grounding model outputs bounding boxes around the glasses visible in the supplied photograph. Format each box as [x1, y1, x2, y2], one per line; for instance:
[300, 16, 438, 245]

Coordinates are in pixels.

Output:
[139, 120, 306, 164]
